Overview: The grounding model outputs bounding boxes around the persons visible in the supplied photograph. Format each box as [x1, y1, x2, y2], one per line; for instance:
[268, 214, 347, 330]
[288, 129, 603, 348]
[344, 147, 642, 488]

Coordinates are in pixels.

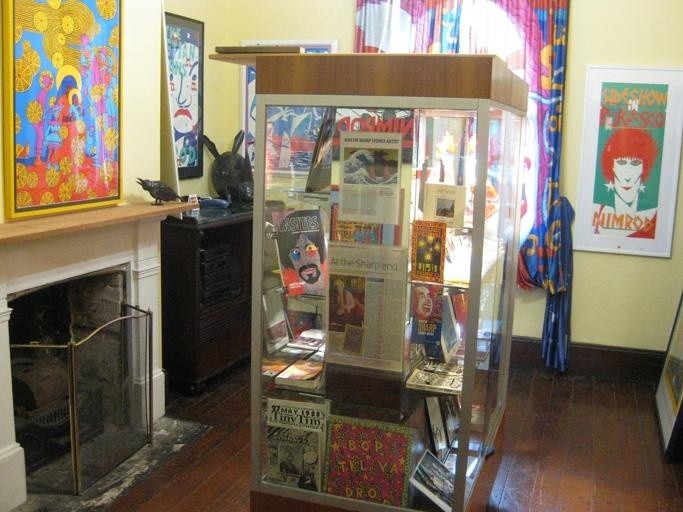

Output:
[593, 128, 657, 230]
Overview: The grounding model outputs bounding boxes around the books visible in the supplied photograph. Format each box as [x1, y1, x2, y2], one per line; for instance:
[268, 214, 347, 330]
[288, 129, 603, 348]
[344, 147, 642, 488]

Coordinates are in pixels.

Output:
[264, 105, 501, 510]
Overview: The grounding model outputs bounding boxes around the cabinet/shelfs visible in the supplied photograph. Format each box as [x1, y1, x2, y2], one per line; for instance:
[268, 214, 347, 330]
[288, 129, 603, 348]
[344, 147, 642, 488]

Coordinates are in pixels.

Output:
[160, 214, 253, 395]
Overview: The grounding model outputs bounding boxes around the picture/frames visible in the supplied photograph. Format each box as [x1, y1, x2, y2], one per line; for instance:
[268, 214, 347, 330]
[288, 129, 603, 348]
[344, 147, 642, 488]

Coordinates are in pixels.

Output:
[164, 11, 204, 180]
[653, 290, 683, 459]
[1, 0, 125, 220]
[572, 66, 682, 258]
[240, 40, 337, 179]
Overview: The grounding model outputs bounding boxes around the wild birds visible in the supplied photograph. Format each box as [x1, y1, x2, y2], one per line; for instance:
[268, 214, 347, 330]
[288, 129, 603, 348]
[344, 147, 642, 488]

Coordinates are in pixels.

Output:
[136, 178, 186, 205]
